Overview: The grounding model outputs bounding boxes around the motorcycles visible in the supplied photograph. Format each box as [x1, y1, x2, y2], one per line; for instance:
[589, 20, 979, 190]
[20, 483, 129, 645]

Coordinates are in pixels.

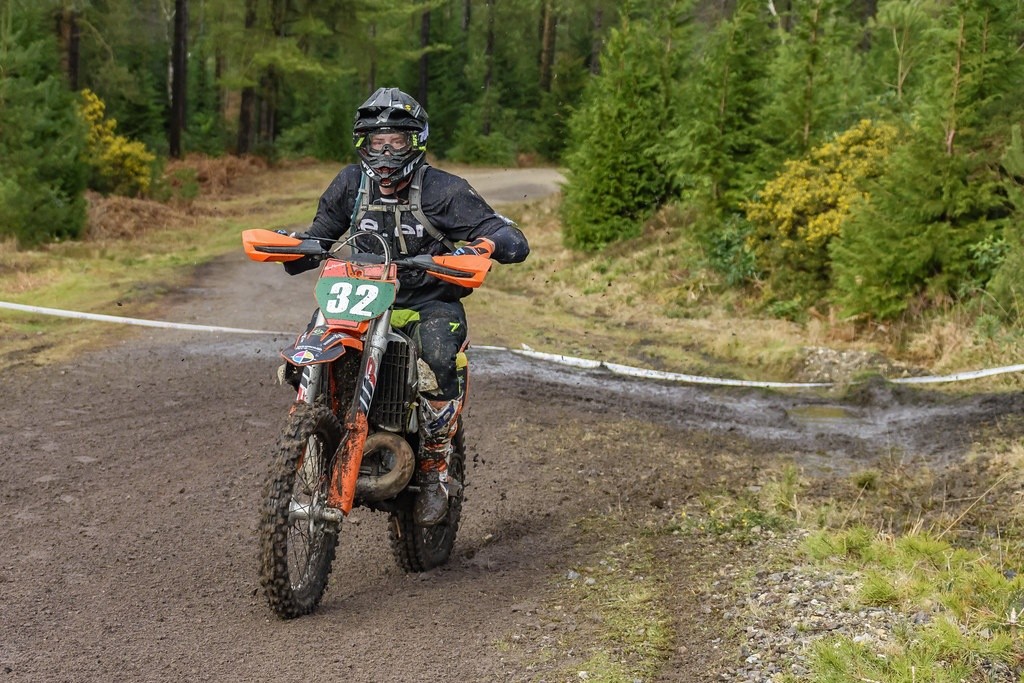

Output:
[241, 228, 491, 620]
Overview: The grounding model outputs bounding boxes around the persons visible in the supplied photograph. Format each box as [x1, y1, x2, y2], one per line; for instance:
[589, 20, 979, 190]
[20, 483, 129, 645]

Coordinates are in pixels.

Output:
[273, 86, 530, 528]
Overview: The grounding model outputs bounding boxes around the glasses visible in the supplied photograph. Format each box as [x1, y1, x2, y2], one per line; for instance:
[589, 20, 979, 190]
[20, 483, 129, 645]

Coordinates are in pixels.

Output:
[360, 129, 420, 157]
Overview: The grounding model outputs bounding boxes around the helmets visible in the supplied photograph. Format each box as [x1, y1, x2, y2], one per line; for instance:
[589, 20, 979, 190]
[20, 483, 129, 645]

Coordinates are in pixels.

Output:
[352, 87, 430, 187]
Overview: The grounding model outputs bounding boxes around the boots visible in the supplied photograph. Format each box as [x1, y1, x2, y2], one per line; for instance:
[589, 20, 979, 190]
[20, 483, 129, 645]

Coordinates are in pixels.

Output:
[413, 389, 464, 528]
[300, 433, 320, 497]
